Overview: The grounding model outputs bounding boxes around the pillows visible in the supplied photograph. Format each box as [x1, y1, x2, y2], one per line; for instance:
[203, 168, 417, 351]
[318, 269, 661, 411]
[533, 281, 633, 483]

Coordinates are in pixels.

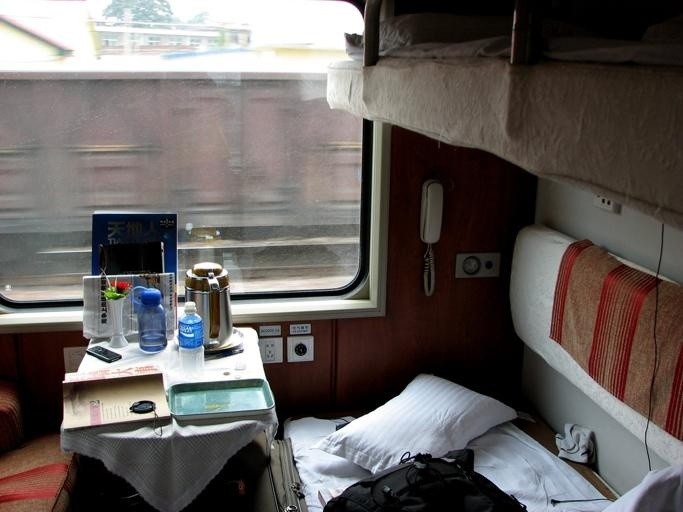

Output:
[309, 373, 518, 474]
[378, 12, 509, 46]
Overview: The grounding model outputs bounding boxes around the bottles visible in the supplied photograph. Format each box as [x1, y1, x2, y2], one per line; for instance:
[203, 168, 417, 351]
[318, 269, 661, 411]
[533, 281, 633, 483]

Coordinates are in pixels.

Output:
[130, 286, 168, 353]
[184, 261, 233, 349]
[177, 301, 204, 381]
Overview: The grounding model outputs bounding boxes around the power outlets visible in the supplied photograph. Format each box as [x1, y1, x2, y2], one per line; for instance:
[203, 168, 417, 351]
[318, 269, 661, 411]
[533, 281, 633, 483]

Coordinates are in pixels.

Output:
[592, 189, 622, 215]
[259, 337, 283, 364]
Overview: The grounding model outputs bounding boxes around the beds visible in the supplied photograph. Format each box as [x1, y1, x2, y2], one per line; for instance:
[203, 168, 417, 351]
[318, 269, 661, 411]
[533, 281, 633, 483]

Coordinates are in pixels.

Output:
[283, 388, 683, 511]
[326, 0, 683, 227]
[0, 375, 92, 511]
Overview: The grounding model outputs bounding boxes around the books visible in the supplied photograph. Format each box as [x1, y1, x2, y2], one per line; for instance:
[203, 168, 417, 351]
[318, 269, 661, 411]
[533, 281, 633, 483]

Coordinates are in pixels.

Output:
[90, 208, 178, 307]
[60, 364, 171, 432]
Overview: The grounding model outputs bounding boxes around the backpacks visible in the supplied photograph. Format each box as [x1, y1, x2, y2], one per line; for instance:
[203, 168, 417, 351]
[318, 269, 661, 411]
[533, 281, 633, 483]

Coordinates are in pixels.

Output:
[323, 449, 528, 512]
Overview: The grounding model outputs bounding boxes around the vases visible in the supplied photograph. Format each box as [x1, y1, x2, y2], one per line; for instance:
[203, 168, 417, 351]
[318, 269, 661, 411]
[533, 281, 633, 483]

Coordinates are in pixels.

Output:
[105, 298, 129, 348]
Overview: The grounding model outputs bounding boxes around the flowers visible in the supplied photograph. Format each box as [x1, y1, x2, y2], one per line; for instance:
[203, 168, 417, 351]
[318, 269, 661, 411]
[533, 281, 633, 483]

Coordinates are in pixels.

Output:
[98, 268, 132, 300]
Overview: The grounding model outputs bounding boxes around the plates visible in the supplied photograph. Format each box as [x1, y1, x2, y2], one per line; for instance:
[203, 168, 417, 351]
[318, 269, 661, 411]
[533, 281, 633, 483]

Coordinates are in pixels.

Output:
[173, 326, 244, 355]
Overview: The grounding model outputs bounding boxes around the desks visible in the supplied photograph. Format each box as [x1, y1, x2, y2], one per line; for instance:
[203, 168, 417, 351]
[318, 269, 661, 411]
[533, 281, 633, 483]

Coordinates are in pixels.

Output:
[60, 326, 273, 511]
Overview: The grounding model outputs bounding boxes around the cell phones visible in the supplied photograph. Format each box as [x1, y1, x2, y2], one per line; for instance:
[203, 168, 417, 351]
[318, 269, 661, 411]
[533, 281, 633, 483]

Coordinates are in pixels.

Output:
[85, 345, 122, 363]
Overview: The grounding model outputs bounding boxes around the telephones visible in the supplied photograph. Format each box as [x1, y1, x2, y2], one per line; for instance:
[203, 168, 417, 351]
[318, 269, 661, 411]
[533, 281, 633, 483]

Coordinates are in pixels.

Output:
[420, 180, 444, 244]
